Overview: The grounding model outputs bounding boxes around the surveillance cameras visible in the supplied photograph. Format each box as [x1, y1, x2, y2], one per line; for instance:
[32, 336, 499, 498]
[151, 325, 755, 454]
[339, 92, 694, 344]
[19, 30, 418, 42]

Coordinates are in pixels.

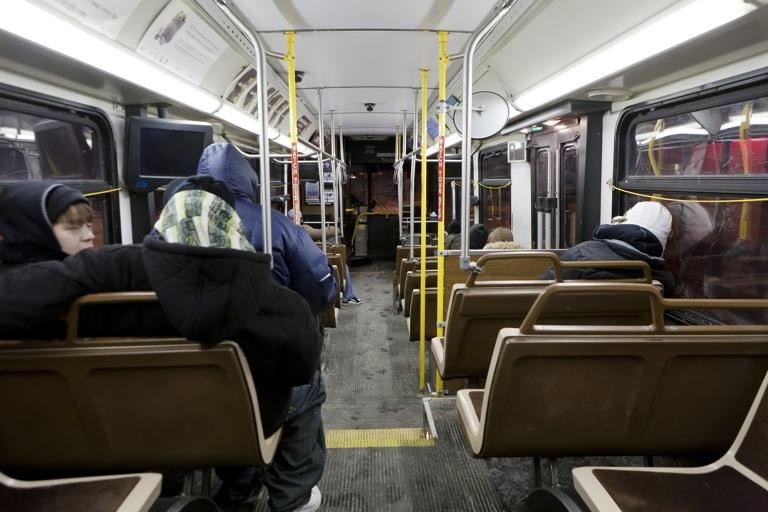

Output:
[367, 135, 374, 139]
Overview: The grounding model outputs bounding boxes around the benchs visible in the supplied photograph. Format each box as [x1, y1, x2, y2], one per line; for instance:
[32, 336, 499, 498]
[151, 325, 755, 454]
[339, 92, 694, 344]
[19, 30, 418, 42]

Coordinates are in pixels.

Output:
[387, 218, 768, 510]
[0, 239, 348, 510]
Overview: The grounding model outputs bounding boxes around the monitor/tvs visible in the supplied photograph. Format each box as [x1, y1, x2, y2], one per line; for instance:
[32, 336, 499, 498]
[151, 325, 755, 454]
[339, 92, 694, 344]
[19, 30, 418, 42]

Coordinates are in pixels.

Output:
[123, 116, 213, 193]
[34, 120, 93, 179]
[299, 164, 319, 182]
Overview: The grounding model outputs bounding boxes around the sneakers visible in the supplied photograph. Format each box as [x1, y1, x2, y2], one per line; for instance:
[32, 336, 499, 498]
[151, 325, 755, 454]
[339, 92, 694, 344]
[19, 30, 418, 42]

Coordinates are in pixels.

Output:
[293, 484, 322, 512]
[342, 297, 364, 305]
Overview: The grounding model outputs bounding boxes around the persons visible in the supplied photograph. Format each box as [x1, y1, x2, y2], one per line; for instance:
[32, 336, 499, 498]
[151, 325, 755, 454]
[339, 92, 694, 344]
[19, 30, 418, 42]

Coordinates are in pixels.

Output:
[0, 177, 95, 260]
[444, 220, 461, 250]
[542, 202, 683, 285]
[149, 143, 340, 311]
[469, 224, 489, 249]
[0, 176, 326, 512]
[288, 208, 364, 305]
[485, 226, 523, 250]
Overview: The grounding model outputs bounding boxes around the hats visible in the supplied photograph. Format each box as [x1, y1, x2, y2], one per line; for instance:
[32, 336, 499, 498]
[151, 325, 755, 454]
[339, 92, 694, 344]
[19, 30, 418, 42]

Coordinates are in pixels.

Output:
[619, 201, 674, 252]
[44, 186, 92, 222]
